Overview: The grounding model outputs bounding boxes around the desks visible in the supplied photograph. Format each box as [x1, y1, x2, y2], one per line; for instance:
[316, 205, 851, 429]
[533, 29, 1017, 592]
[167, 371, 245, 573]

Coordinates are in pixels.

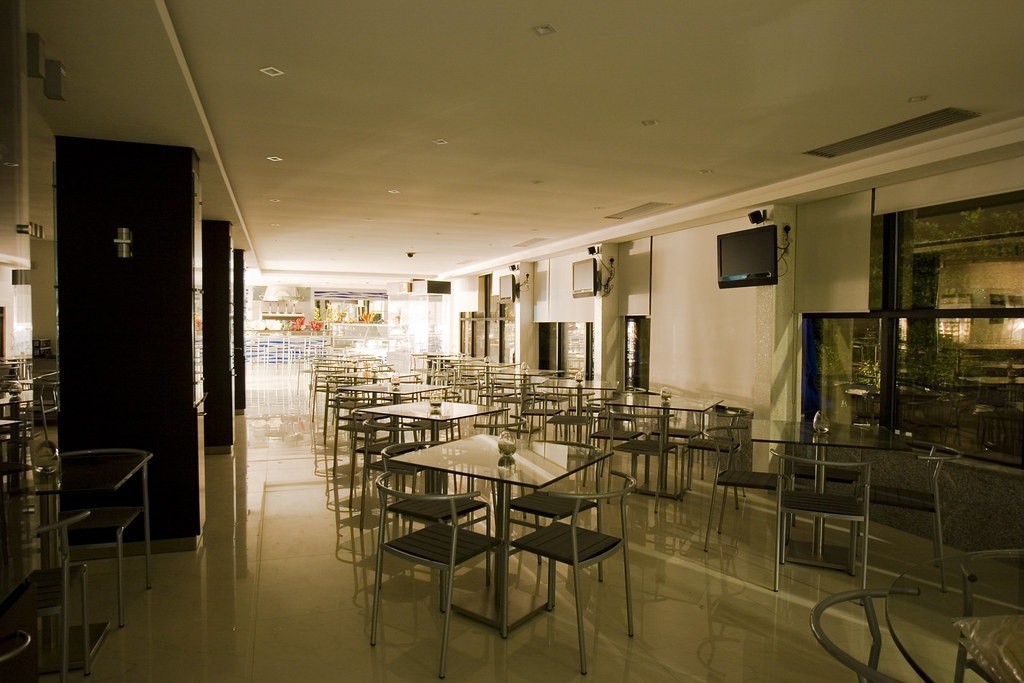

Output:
[480, 369, 565, 433]
[0, 454, 152, 677]
[885, 547, 1024, 682]
[329, 370, 421, 442]
[0, 389, 43, 439]
[453, 362, 521, 391]
[355, 399, 511, 496]
[750, 418, 915, 573]
[314, 363, 396, 399]
[430, 358, 484, 385]
[338, 382, 452, 454]
[539, 378, 620, 442]
[387, 432, 616, 633]
[0, 419, 35, 494]
[603, 393, 724, 498]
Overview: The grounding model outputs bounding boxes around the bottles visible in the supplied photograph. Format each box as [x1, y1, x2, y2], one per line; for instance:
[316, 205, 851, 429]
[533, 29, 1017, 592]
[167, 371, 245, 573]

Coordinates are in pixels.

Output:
[8, 381, 22, 399]
[391, 375, 400, 389]
[352, 358, 358, 367]
[575, 371, 584, 384]
[813, 411, 830, 434]
[457, 352, 463, 359]
[371, 365, 379, 377]
[484, 356, 490, 364]
[32, 440, 59, 474]
[498, 430, 517, 458]
[521, 362, 528, 371]
[660, 387, 671, 402]
[430, 391, 443, 408]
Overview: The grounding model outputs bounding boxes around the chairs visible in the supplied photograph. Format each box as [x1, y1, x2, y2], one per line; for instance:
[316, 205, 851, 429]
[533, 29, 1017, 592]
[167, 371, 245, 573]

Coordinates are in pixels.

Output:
[242, 333, 1024, 683]
[0, 383, 153, 682]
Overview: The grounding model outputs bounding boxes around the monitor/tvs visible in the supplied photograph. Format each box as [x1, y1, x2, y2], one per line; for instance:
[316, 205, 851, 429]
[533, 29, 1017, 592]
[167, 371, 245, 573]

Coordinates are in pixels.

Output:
[572, 259, 597, 298]
[717, 224, 778, 289]
[499, 274, 516, 304]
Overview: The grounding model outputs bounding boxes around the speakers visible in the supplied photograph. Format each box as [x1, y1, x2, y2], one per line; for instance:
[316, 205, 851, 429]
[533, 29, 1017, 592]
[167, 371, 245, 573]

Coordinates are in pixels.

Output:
[44, 59, 67, 102]
[25, 33, 46, 79]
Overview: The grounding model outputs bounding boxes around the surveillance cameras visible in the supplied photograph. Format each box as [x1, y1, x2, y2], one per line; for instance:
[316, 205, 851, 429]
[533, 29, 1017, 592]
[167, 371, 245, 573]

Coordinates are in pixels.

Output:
[406, 253, 413, 258]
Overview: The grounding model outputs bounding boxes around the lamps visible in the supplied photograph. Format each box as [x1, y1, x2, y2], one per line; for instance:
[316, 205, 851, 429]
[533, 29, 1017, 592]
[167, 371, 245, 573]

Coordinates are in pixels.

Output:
[115, 227, 133, 259]
[509, 264, 519, 271]
[586, 246, 599, 255]
[748, 210, 767, 225]
